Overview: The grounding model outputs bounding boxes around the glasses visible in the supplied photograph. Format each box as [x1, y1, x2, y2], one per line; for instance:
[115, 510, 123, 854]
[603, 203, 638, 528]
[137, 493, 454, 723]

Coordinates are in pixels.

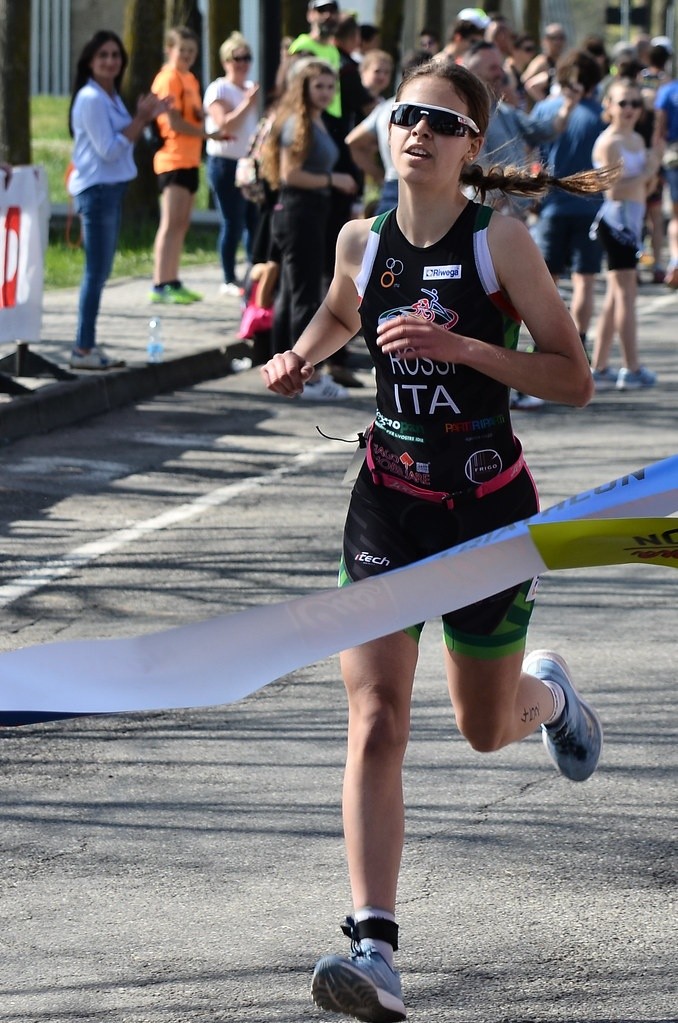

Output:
[388, 102, 480, 137]
[226, 53, 251, 63]
[617, 98, 643, 109]
[520, 45, 536, 54]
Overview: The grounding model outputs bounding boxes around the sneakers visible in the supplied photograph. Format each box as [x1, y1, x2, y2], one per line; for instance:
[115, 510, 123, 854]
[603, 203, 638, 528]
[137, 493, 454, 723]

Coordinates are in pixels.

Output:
[615, 367, 657, 389]
[310, 942, 406, 1022]
[70, 346, 126, 370]
[297, 374, 349, 402]
[589, 367, 619, 391]
[150, 282, 203, 305]
[523, 650, 604, 781]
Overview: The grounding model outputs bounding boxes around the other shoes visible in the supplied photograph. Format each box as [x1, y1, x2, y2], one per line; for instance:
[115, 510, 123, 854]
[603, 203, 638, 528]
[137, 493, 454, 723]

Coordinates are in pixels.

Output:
[221, 280, 244, 298]
[321, 363, 364, 387]
[667, 267, 678, 286]
[636, 268, 665, 283]
[509, 390, 544, 409]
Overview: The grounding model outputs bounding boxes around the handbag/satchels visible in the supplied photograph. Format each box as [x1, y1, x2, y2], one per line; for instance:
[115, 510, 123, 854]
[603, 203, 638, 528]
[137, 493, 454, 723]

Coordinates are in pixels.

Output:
[235, 119, 266, 203]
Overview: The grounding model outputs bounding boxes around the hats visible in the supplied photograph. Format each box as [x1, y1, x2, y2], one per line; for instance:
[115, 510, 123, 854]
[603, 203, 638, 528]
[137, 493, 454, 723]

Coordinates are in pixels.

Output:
[458, 8, 490, 29]
[308, 0, 337, 9]
[651, 36, 674, 55]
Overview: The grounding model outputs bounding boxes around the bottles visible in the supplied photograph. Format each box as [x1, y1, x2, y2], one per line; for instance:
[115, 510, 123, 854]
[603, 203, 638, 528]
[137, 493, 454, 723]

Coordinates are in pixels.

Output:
[147, 315, 163, 362]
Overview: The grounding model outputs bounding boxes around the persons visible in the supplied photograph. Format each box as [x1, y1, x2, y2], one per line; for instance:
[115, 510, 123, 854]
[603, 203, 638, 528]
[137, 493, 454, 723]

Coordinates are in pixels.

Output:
[151, 24, 236, 304]
[204, 31, 259, 293]
[68, 30, 173, 368]
[243, 0, 678, 405]
[259, 62, 603, 1023]
